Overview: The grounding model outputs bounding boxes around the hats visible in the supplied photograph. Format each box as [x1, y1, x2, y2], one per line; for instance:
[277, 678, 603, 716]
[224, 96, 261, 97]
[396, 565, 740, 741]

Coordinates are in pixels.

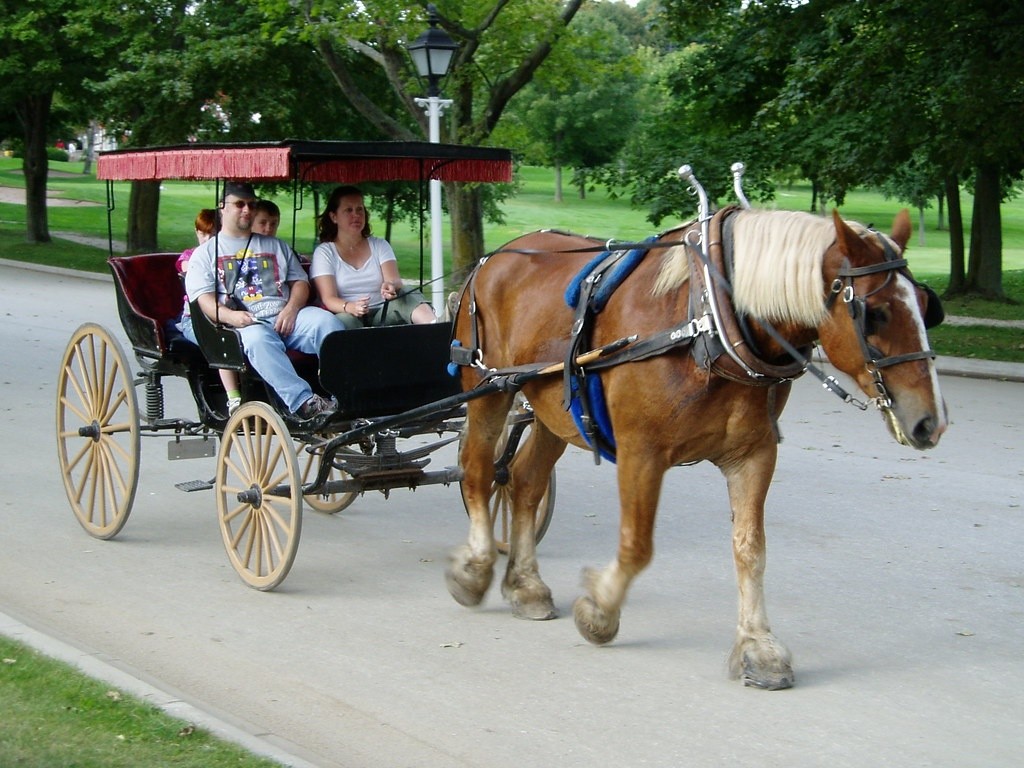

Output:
[219, 181, 260, 200]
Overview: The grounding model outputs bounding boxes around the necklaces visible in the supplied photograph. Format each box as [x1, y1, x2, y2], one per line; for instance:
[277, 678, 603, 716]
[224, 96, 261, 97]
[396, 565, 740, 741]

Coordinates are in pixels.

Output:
[344, 243, 359, 250]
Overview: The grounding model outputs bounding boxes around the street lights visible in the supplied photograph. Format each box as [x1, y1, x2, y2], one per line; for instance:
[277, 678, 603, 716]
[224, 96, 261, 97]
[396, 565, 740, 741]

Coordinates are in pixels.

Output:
[406, 3, 464, 320]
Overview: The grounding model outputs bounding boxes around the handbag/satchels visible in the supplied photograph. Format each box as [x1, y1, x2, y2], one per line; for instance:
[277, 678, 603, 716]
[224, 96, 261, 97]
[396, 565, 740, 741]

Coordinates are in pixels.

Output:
[220, 296, 247, 329]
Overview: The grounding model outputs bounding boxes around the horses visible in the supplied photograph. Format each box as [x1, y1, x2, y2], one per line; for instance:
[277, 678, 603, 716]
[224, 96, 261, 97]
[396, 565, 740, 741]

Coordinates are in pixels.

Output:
[443, 205, 949, 692]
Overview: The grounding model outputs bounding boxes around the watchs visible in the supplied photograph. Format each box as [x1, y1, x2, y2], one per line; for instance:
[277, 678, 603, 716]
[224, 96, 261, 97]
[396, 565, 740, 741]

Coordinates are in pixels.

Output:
[343, 301, 349, 313]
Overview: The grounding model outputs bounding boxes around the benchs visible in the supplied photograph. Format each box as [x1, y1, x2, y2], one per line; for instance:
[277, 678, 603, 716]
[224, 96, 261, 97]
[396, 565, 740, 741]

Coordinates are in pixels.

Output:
[106, 250, 334, 372]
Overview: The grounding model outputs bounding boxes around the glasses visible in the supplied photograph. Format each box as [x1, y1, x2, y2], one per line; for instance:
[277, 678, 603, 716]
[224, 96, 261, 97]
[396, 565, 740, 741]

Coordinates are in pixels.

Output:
[223, 200, 258, 211]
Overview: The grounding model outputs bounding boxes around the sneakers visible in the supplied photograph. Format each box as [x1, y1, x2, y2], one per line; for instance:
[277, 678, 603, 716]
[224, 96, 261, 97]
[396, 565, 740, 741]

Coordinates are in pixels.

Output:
[436, 291, 459, 323]
[226, 397, 241, 417]
[297, 393, 335, 420]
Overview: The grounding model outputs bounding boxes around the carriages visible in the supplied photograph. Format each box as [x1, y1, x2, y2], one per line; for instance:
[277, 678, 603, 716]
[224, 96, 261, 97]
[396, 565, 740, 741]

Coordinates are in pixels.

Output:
[54, 138, 946, 691]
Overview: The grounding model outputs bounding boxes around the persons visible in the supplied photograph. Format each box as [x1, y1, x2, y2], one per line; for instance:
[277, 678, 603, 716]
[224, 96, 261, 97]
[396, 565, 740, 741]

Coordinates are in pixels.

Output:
[175, 179, 458, 419]
[56, 138, 65, 150]
[68, 140, 76, 162]
